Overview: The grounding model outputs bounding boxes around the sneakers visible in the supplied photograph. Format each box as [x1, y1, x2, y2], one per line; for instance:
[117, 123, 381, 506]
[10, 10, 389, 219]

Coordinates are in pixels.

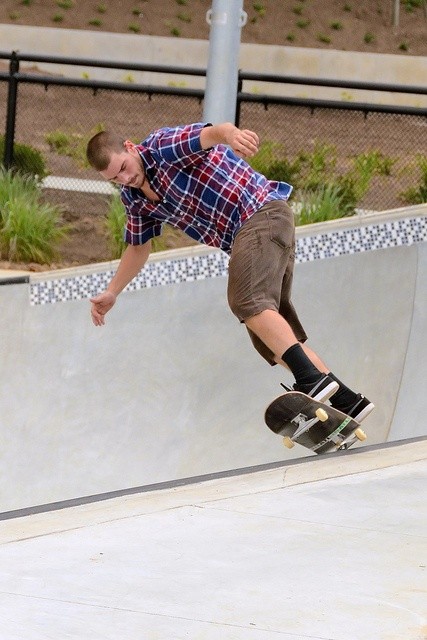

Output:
[280, 372, 340, 404]
[331, 392, 376, 425]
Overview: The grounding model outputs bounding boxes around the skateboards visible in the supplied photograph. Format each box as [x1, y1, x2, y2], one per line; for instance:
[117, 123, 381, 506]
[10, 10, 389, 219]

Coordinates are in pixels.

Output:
[265, 390, 367, 454]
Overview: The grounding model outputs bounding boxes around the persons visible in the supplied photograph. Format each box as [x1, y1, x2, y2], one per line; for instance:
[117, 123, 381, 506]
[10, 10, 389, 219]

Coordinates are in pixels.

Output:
[86, 120, 376, 424]
